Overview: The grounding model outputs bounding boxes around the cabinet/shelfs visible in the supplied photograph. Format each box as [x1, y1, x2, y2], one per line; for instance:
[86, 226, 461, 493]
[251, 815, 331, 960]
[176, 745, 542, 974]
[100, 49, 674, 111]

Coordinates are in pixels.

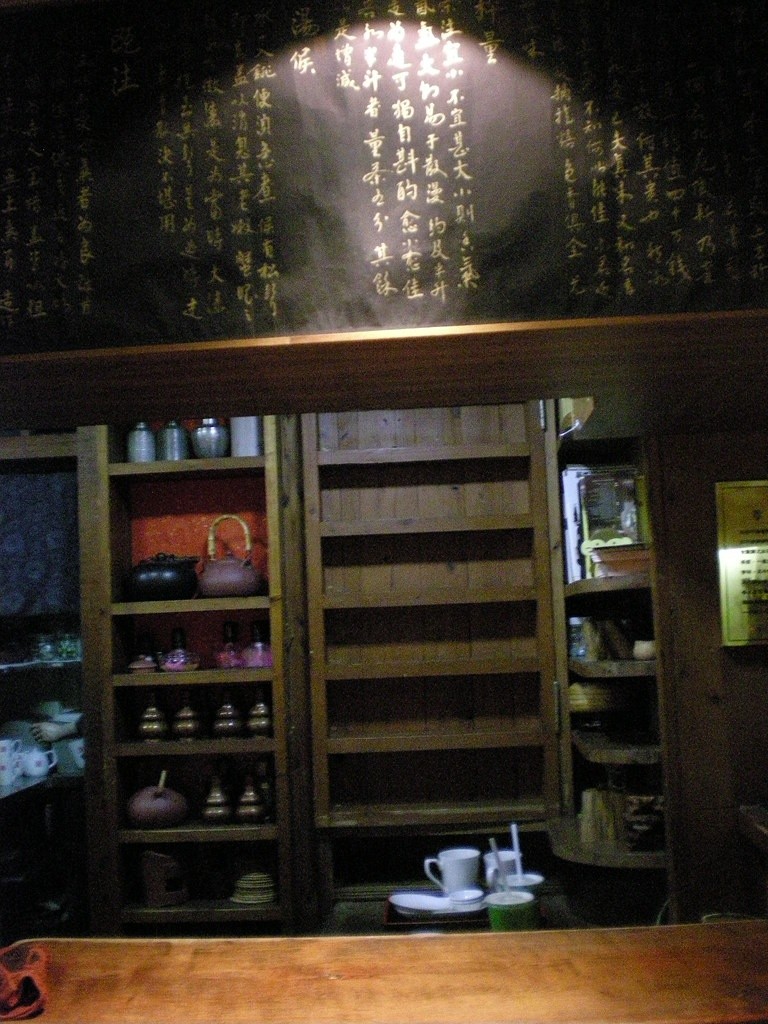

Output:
[1, 399, 767, 919]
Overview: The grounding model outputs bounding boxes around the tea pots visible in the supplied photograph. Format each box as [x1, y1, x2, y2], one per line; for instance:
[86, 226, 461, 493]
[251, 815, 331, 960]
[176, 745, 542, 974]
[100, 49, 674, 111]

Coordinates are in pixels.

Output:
[199, 514, 263, 596]
[127, 553, 201, 601]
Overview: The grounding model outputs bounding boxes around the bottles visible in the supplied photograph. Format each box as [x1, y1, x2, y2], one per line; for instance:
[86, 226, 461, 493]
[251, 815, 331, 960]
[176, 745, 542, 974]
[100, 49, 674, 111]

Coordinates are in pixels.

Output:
[125, 621, 273, 675]
[230, 872, 275, 903]
[139, 689, 271, 742]
[578, 779, 633, 852]
[126, 761, 276, 828]
[128, 417, 228, 463]
[569, 614, 637, 663]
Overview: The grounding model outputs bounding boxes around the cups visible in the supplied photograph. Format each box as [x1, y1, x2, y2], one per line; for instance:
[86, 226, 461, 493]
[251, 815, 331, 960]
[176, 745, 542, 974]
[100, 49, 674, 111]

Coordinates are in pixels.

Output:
[56, 634, 81, 660]
[0, 700, 87, 788]
[230, 416, 260, 457]
[632, 641, 655, 659]
[29, 634, 57, 661]
[423, 847, 546, 931]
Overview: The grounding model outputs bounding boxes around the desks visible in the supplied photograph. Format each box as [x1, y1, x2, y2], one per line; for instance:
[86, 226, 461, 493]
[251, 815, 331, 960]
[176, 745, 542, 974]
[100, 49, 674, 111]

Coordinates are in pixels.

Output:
[0, 921, 768, 1024]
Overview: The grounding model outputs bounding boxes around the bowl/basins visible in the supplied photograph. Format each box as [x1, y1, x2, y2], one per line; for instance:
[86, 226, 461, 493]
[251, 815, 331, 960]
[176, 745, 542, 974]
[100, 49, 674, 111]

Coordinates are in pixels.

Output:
[388, 888, 487, 919]
[594, 544, 649, 575]
